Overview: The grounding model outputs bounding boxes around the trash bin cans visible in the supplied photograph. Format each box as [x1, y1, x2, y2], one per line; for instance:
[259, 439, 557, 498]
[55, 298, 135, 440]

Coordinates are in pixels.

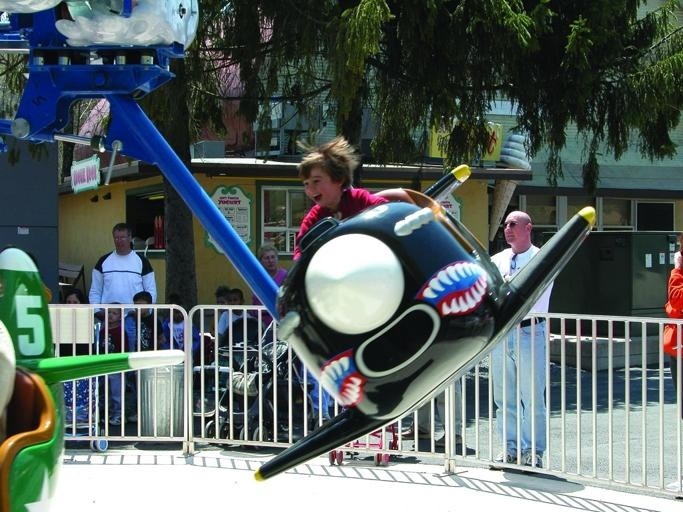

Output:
[134, 363, 184, 450]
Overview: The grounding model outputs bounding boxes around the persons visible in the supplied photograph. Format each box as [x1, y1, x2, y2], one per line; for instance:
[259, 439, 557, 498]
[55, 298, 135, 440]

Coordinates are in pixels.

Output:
[484, 211, 552, 473]
[251, 244, 287, 332]
[399, 374, 462, 447]
[59, 223, 256, 426]
[658, 237, 683, 386]
[305, 370, 332, 427]
[292, 136, 384, 261]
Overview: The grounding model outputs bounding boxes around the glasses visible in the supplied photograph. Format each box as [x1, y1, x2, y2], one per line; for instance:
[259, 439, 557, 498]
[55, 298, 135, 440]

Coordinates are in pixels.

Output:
[502, 222, 525, 229]
[113, 236, 130, 241]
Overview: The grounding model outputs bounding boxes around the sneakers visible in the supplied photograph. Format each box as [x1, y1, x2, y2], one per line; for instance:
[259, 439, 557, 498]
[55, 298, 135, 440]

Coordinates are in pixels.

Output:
[432, 432, 463, 448]
[488, 447, 543, 474]
[393, 427, 432, 441]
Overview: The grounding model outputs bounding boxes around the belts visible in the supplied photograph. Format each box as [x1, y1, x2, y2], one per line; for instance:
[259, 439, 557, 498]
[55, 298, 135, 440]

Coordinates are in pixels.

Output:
[518, 316, 546, 329]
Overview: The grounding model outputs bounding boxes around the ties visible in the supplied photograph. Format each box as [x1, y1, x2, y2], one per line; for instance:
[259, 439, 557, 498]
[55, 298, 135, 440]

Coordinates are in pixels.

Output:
[508, 253, 518, 272]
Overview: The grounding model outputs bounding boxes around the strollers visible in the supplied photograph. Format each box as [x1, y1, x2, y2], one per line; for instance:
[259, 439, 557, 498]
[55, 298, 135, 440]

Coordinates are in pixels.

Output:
[184, 319, 400, 466]
